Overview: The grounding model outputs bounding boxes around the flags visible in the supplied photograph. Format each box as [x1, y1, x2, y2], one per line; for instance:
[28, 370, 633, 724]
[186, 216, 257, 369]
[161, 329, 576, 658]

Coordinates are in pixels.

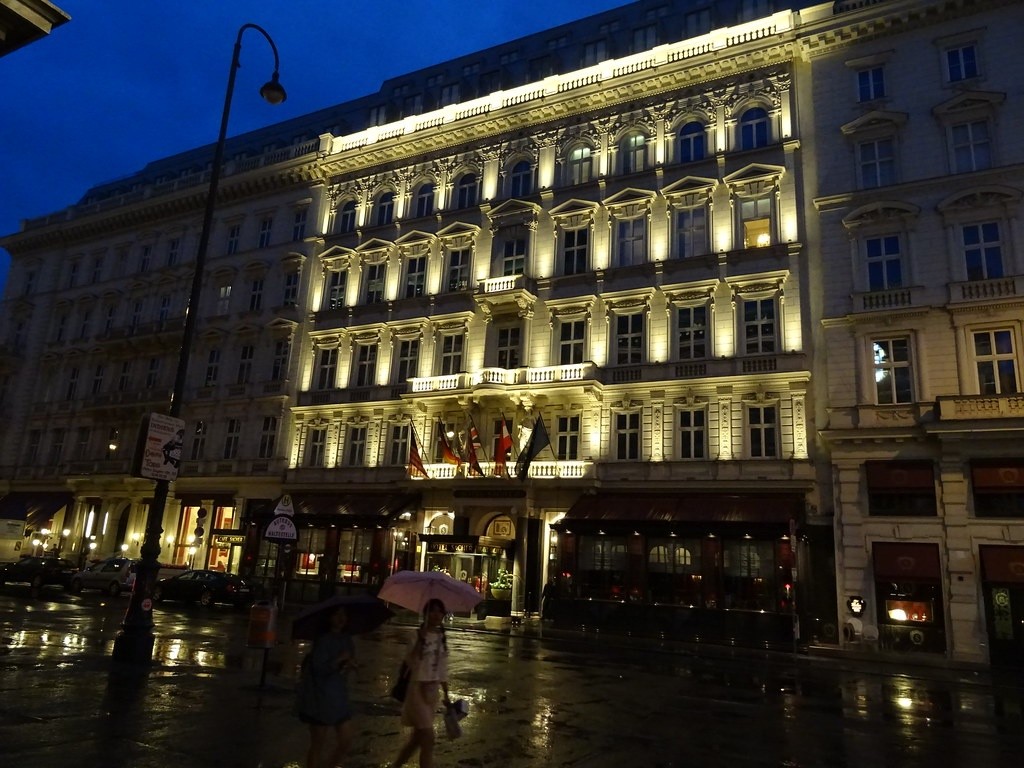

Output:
[410, 427, 430, 478]
[436, 424, 465, 466]
[494, 416, 513, 478]
[466, 419, 486, 477]
[514, 417, 550, 483]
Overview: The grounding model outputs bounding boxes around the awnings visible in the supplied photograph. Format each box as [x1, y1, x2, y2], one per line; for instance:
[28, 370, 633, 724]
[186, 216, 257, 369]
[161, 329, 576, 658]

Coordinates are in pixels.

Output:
[873, 544, 941, 585]
[0, 491, 73, 520]
[561, 493, 799, 522]
[865, 459, 935, 495]
[980, 546, 1024, 586]
[255, 490, 416, 517]
[971, 458, 1024, 494]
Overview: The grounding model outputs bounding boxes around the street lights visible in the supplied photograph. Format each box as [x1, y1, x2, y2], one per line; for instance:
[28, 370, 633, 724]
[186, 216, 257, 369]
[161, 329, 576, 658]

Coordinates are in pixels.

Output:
[112, 21, 291, 667]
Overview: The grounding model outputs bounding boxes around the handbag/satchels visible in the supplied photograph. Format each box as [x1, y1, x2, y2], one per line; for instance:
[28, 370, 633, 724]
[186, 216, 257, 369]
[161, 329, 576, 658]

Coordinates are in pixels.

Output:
[390, 629, 423, 703]
[443, 699, 469, 741]
[335, 660, 372, 698]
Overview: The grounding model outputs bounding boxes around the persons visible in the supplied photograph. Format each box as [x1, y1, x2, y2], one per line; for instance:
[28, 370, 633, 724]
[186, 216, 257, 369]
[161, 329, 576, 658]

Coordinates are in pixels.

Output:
[297, 608, 354, 768]
[389, 600, 451, 768]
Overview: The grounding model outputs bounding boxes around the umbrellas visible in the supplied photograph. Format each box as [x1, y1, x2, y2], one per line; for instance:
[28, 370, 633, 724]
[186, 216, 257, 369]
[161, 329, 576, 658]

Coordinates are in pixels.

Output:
[290, 593, 394, 640]
[377, 570, 484, 658]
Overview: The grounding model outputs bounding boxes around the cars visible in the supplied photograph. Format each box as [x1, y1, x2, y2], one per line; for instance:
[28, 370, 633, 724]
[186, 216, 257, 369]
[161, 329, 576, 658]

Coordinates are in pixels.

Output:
[3, 555, 76, 589]
[152, 567, 252, 609]
[70, 557, 141, 597]
